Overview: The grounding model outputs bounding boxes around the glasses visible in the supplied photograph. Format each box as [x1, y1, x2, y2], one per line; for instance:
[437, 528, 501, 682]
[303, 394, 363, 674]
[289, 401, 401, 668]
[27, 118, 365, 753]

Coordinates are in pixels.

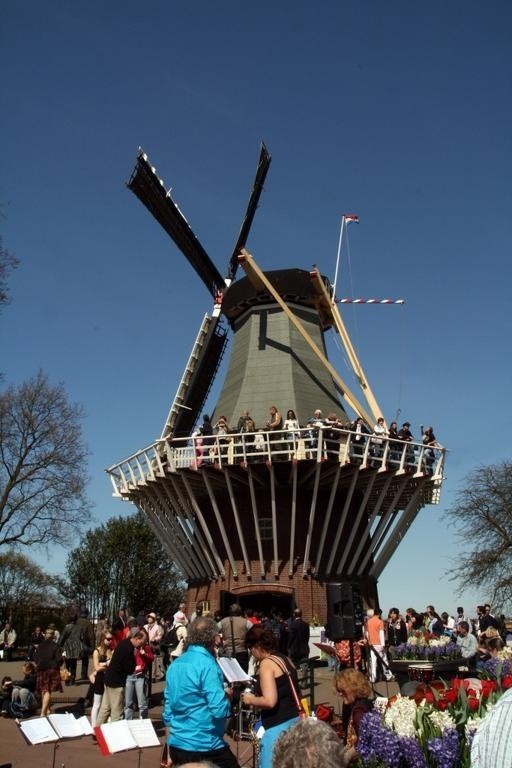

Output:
[104, 636, 113, 643]
[484, 608, 490, 612]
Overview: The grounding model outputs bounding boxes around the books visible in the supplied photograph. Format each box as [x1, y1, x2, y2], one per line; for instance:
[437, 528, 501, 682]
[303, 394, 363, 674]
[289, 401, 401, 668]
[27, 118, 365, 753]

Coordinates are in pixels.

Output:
[17, 710, 82, 746]
[101, 719, 162, 754]
[216, 656, 251, 682]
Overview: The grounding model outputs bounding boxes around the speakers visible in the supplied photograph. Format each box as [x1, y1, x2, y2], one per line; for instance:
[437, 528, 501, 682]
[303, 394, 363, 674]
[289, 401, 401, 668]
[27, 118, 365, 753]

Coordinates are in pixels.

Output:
[326, 583, 362, 641]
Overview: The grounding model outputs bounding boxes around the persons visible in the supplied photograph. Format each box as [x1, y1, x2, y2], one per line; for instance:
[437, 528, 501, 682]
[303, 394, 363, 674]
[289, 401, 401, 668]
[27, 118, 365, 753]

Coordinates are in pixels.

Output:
[214, 417, 229, 455]
[192, 427, 203, 469]
[241, 625, 303, 767]
[93, 630, 146, 742]
[470, 686, 511, 767]
[92, 631, 113, 726]
[113, 611, 127, 628]
[441, 611, 457, 635]
[238, 411, 253, 438]
[269, 718, 344, 768]
[399, 421, 414, 460]
[427, 611, 443, 637]
[290, 609, 309, 664]
[363, 610, 375, 654]
[421, 427, 437, 479]
[213, 604, 254, 674]
[458, 621, 477, 666]
[37, 632, 66, 718]
[240, 421, 253, 462]
[0, 677, 14, 716]
[167, 616, 186, 660]
[163, 618, 244, 768]
[128, 620, 141, 638]
[255, 429, 266, 460]
[192, 603, 207, 618]
[125, 646, 154, 720]
[6, 663, 39, 715]
[75, 608, 94, 679]
[322, 414, 342, 446]
[267, 406, 282, 459]
[349, 418, 372, 456]
[424, 606, 437, 629]
[44, 624, 58, 644]
[489, 639, 505, 663]
[95, 615, 107, 646]
[259, 610, 289, 626]
[370, 610, 394, 681]
[307, 410, 326, 428]
[56, 615, 83, 687]
[191, 604, 203, 621]
[410, 613, 424, 636]
[27, 627, 42, 661]
[374, 418, 390, 465]
[477, 626, 498, 658]
[335, 669, 382, 765]
[303, 424, 318, 457]
[244, 609, 258, 623]
[476, 606, 493, 634]
[455, 607, 472, 635]
[200, 415, 213, 455]
[390, 609, 407, 647]
[388, 424, 402, 453]
[144, 613, 163, 683]
[407, 609, 419, 636]
[173, 604, 188, 623]
[282, 411, 301, 459]
[485, 604, 490, 616]
[2, 623, 17, 662]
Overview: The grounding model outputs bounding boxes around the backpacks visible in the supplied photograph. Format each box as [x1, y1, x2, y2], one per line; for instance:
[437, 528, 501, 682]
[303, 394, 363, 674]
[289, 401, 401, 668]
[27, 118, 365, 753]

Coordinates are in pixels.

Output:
[165, 625, 185, 648]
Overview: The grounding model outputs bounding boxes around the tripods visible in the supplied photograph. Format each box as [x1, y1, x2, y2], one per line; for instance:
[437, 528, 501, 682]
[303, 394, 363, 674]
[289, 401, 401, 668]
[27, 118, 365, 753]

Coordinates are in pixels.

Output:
[135, 747, 144, 768]
[51, 745, 60, 768]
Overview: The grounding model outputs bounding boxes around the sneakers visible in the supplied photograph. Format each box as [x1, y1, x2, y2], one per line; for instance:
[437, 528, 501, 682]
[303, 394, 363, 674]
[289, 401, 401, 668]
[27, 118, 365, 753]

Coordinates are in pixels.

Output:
[385, 675, 398, 682]
[370, 678, 376, 684]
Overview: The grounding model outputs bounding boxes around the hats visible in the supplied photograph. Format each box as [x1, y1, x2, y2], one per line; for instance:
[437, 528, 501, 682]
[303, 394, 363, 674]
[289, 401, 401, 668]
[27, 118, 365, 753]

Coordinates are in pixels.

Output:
[145, 611, 157, 623]
[172, 612, 186, 624]
